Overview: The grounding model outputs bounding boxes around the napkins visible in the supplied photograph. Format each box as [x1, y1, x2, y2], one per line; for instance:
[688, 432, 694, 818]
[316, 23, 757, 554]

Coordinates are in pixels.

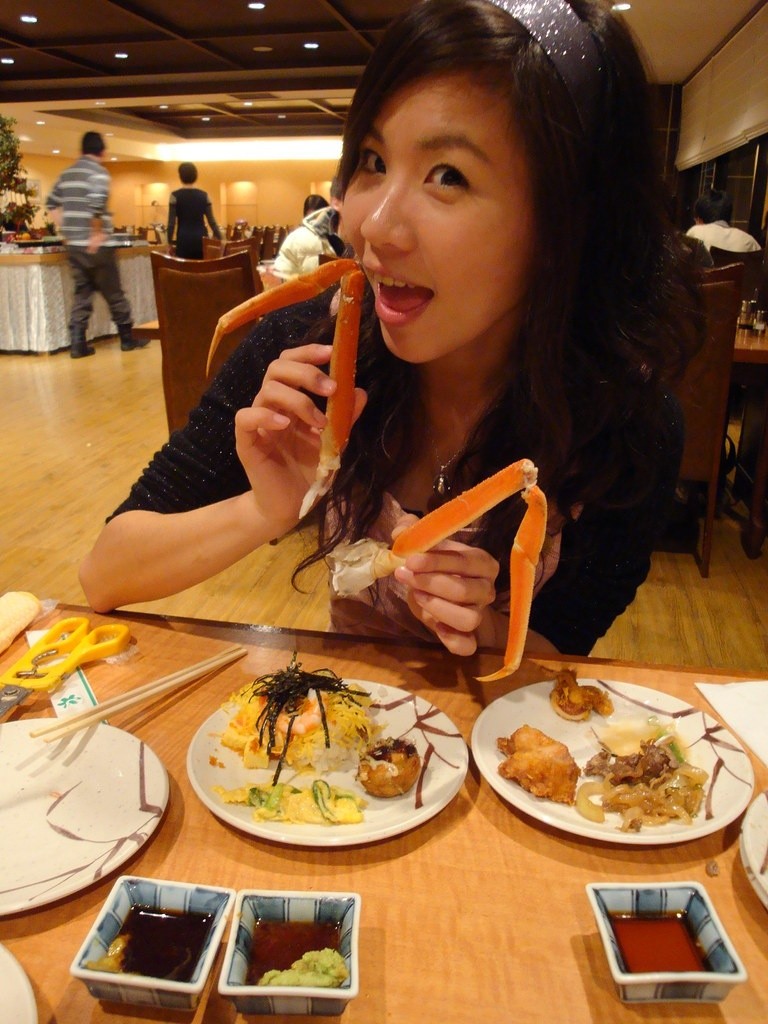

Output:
[696, 680, 768, 769]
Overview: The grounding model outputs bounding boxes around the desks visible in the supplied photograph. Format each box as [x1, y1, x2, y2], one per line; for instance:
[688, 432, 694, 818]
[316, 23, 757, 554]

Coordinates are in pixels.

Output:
[0, 603, 768, 1024]
[256, 264, 286, 291]
[732, 312, 768, 365]
[0, 241, 168, 357]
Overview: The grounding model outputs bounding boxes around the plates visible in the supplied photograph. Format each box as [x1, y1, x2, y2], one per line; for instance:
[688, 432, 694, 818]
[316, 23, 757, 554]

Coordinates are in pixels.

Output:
[471, 679, 755, 844]
[739, 789, 768, 910]
[186, 679, 468, 846]
[0, 943, 38, 1024]
[0, 718, 169, 916]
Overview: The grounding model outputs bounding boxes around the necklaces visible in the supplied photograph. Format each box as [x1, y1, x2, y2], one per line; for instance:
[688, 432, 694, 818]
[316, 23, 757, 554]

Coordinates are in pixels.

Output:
[422, 405, 463, 495]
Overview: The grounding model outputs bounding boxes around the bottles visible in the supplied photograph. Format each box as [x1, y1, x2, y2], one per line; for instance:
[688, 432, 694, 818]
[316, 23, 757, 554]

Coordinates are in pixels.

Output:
[754, 310, 766, 334]
[739, 300, 756, 328]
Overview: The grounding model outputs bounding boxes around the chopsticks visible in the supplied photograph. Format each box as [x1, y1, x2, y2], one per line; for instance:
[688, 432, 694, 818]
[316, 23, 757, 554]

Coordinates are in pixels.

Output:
[29, 643, 248, 743]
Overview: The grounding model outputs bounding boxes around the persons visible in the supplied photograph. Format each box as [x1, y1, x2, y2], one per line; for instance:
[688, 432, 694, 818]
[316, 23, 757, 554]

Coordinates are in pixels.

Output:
[79, 0, 685, 657]
[273, 194, 345, 278]
[167, 161, 223, 261]
[43, 131, 151, 358]
[657, 188, 768, 267]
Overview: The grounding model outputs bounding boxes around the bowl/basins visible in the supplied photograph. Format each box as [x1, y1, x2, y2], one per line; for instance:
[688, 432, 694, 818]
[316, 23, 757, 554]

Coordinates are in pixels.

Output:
[70, 875, 237, 1010]
[586, 881, 747, 1004]
[217, 889, 361, 1016]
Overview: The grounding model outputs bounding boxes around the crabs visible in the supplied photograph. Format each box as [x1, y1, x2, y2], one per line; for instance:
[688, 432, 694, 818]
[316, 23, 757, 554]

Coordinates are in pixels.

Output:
[333, 458, 549, 683]
[204, 256, 365, 511]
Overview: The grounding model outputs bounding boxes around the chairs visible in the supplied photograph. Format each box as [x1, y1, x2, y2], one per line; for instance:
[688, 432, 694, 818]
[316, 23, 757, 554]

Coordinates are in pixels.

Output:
[118, 223, 295, 440]
[676, 243, 767, 579]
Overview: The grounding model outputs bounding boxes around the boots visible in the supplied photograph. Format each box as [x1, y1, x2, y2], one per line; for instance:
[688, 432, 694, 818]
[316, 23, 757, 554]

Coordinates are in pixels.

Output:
[117, 323, 151, 351]
[71, 333, 95, 358]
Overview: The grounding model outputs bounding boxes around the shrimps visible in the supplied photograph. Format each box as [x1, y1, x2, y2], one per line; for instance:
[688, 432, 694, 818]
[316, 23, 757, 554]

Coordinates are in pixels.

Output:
[258, 685, 329, 736]
